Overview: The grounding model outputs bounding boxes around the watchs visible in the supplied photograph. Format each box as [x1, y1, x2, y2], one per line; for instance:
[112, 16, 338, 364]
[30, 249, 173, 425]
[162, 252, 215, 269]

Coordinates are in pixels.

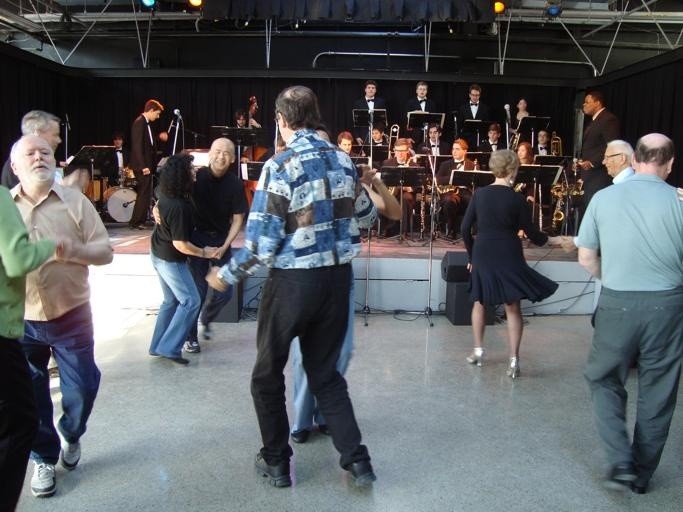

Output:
[214, 271, 227, 284]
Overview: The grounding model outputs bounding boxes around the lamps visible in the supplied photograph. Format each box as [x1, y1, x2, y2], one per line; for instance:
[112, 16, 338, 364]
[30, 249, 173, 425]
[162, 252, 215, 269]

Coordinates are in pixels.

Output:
[542, 0, 562, 18]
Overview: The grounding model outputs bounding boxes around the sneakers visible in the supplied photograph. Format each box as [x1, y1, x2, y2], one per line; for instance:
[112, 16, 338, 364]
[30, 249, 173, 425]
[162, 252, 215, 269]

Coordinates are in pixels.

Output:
[58, 434, 82, 470]
[184, 338, 202, 354]
[27, 460, 57, 498]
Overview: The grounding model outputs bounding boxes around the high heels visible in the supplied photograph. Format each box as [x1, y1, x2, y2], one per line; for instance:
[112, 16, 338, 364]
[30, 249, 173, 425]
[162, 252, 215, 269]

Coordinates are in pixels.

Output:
[504, 353, 524, 379]
[463, 346, 488, 368]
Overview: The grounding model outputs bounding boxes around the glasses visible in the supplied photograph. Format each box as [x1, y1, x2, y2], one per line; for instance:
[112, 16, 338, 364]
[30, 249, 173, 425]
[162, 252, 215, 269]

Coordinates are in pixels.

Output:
[603, 153, 623, 160]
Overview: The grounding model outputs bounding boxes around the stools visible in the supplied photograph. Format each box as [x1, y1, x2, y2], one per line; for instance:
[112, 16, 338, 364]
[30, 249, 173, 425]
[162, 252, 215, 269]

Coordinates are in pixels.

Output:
[371, 200, 579, 244]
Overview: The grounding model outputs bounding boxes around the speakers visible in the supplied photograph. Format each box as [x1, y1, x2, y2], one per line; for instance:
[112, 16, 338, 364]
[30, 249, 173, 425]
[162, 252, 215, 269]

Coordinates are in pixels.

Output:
[441, 251, 479, 280]
[209, 279, 244, 323]
[446, 283, 494, 326]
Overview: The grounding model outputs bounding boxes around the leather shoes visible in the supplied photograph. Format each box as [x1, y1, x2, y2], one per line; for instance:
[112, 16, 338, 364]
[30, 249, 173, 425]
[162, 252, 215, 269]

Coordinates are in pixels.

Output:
[290, 429, 310, 444]
[253, 449, 294, 487]
[169, 354, 192, 366]
[348, 458, 379, 489]
[319, 421, 331, 435]
[597, 462, 636, 493]
[131, 222, 153, 230]
[627, 482, 649, 495]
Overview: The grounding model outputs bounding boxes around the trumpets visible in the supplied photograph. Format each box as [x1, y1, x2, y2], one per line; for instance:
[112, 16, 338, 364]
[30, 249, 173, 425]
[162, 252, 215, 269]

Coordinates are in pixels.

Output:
[551, 131, 584, 228]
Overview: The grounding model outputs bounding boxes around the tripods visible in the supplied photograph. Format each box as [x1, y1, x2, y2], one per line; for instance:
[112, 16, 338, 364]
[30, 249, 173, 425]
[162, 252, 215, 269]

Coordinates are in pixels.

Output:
[355, 229, 399, 325]
[361, 201, 476, 246]
[395, 194, 446, 327]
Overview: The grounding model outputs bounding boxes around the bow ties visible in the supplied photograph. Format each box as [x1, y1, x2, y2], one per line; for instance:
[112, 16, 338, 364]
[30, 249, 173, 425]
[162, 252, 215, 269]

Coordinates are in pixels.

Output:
[489, 143, 497, 147]
[430, 144, 440, 148]
[397, 162, 404, 166]
[469, 102, 478, 107]
[365, 98, 374, 103]
[538, 145, 549, 151]
[374, 141, 382, 146]
[418, 98, 426, 104]
[453, 160, 465, 167]
[115, 149, 122, 154]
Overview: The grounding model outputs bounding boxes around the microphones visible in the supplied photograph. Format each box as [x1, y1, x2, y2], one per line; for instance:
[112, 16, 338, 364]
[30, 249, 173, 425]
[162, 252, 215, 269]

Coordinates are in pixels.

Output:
[368, 109, 375, 123]
[505, 104, 513, 121]
[174, 108, 180, 119]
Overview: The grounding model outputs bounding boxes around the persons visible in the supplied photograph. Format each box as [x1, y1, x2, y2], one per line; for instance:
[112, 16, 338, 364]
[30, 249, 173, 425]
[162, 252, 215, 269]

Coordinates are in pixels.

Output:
[459, 149, 549, 379]
[231, 109, 261, 177]
[508, 97, 534, 147]
[676, 187, 683, 202]
[511, 142, 535, 241]
[205, 126, 403, 442]
[336, 131, 361, 158]
[533, 130, 552, 155]
[403, 82, 436, 139]
[382, 138, 420, 239]
[457, 85, 491, 144]
[0, 111, 62, 189]
[573, 133, 682, 495]
[151, 136, 244, 355]
[147, 154, 218, 365]
[477, 122, 505, 186]
[351, 81, 387, 146]
[128, 99, 168, 231]
[0, 180, 61, 510]
[415, 124, 450, 155]
[108, 131, 128, 187]
[575, 92, 620, 237]
[205, 86, 378, 489]
[591, 139, 637, 368]
[436, 140, 480, 240]
[370, 122, 388, 146]
[8, 135, 113, 497]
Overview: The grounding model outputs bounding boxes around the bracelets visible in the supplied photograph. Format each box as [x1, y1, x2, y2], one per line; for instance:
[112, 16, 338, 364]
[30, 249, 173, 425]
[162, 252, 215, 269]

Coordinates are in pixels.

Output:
[201, 245, 208, 259]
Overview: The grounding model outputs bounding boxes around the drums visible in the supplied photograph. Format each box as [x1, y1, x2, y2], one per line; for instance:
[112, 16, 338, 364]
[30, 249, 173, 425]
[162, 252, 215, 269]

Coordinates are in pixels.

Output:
[83, 177, 137, 223]
